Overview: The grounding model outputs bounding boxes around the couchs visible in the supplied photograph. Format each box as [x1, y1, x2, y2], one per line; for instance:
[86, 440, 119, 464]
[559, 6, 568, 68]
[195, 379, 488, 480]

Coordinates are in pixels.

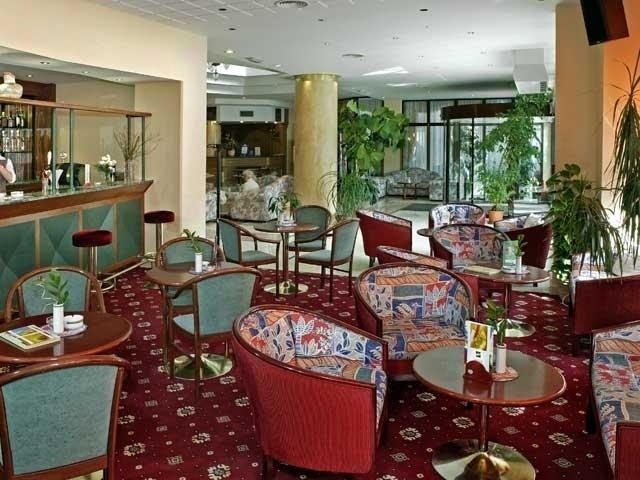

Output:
[230, 174, 294, 223]
[569, 255, 640, 348]
[387, 168, 443, 201]
[223, 175, 280, 193]
[584, 319, 640, 480]
[204, 182, 217, 223]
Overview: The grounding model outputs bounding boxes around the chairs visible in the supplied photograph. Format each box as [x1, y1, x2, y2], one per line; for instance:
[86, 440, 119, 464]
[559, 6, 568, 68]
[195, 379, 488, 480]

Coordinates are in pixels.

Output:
[429, 203, 485, 229]
[217, 218, 281, 298]
[294, 218, 361, 303]
[5, 266, 106, 324]
[232, 304, 390, 480]
[356, 209, 413, 268]
[494, 214, 553, 287]
[162, 268, 264, 397]
[376, 245, 449, 270]
[286, 206, 331, 294]
[354, 263, 474, 399]
[156, 237, 225, 266]
[0, 355, 132, 480]
[432, 223, 511, 270]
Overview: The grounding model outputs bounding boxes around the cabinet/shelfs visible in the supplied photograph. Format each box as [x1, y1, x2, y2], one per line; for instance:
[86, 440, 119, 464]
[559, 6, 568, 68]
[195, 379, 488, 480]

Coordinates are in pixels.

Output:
[222, 157, 286, 187]
[0, 77, 56, 196]
[0, 97, 154, 321]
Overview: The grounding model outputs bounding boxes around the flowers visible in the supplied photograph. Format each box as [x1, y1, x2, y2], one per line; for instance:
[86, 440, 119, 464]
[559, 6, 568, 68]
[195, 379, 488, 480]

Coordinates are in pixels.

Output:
[92, 155, 117, 179]
[183, 229, 203, 272]
[34, 271, 70, 334]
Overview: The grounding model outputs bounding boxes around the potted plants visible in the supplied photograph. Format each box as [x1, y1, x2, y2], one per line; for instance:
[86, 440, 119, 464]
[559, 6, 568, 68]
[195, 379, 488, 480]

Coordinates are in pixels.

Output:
[267, 190, 303, 224]
[476, 168, 522, 224]
[513, 235, 528, 274]
[224, 137, 238, 156]
[112, 123, 161, 181]
[485, 300, 519, 372]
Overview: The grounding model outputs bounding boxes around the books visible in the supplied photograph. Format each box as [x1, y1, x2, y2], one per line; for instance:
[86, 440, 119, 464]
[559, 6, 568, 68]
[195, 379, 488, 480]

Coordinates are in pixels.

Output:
[0, 324, 60, 350]
[464, 321, 494, 374]
[464, 265, 501, 275]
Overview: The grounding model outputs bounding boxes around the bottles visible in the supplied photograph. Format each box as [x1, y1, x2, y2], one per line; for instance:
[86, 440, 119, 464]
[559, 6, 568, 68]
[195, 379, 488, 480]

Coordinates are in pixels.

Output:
[10, 130, 15, 151]
[8, 111, 14, 128]
[21, 111, 24, 128]
[0, 131, 6, 151]
[21, 137, 25, 151]
[6, 131, 10, 151]
[15, 108, 20, 127]
[1, 112, 8, 127]
[16, 130, 21, 151]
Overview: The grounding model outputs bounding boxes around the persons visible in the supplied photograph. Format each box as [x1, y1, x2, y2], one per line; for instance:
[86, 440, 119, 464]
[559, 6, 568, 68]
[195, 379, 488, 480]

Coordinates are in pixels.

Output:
[242, 171, 259, 195]
[0, 154, 17, 196]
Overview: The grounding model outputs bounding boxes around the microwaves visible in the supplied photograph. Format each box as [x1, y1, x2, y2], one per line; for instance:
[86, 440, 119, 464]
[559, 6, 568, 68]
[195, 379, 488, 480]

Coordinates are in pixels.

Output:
[49, 162, 96, 189]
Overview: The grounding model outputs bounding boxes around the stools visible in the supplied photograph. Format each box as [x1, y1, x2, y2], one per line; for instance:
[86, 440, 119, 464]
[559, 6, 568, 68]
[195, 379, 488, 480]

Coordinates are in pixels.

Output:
[72, 230, 116, 293]
[145, 211, 174, 260]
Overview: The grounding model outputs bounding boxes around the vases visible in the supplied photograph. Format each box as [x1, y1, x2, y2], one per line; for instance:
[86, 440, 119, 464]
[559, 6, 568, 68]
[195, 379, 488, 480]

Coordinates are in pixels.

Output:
[103, 176, 110, 184]
